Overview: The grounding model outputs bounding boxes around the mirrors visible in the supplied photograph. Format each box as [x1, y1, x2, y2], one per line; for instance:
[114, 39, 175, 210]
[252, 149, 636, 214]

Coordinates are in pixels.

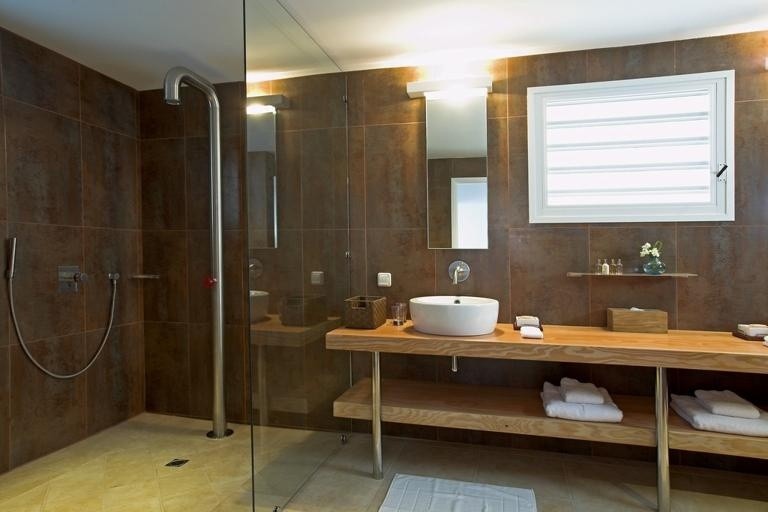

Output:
[423, 91, 492, 252]
[243, 104, 281, 251]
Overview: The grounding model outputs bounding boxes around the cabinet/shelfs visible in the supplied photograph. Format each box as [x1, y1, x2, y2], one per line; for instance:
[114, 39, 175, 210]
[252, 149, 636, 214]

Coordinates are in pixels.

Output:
[565, 269, 698, 281]
[251, 316, 338, 461]
[322, 316, 767, 511]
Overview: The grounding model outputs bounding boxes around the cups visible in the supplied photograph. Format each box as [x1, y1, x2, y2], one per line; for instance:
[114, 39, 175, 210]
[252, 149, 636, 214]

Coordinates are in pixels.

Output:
[391, 303, 408, 326]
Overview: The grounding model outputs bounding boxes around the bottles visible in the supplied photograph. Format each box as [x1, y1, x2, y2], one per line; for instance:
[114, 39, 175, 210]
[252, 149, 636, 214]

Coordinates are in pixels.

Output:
[595, 258, 623, 276]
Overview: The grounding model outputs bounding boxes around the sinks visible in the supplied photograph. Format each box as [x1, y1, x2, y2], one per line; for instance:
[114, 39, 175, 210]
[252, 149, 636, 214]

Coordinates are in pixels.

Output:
[409, 294, 500, 337]
[250, 289, 269, 325]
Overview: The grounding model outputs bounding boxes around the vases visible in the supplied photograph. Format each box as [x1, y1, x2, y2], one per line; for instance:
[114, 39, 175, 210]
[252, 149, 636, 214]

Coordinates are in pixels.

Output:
[642, 255, 666, 274]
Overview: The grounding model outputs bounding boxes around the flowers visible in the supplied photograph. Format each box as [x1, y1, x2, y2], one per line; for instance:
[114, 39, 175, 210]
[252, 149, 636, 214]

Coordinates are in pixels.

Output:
[640, 241, 664, 259]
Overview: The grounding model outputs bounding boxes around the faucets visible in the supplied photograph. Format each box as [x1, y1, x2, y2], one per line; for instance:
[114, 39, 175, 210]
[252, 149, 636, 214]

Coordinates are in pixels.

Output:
[451, 266, 462, 285]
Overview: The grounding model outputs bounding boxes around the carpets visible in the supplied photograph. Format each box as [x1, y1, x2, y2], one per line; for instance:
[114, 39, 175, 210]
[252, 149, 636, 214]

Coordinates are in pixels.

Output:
[378, 471, 536, 511]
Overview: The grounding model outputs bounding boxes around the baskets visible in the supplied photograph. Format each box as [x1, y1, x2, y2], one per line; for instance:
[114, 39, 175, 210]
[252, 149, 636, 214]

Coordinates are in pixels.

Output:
[344, 295, 386, 329]
[279, 293, 329, 328]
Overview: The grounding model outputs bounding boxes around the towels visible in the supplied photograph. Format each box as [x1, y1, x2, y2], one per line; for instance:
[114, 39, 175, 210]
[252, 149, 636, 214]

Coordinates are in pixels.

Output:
[536, 372, 623, 423]
[666, 383, 768, 436]
